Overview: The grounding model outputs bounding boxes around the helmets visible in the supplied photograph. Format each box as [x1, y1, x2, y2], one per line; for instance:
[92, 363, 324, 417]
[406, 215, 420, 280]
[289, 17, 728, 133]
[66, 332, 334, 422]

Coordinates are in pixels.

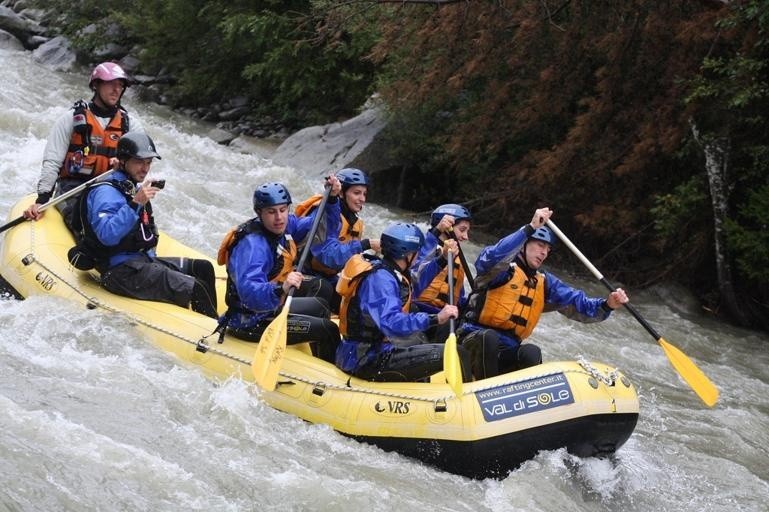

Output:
[335, 168, 371, 193]
[431, 203, 473, 227]
[88, 62, 133, 90]
[253, 182, 292, 210]
[115, 131, 162, 160]
[380, 221, 425, 258]
[530, 225, 556, 246]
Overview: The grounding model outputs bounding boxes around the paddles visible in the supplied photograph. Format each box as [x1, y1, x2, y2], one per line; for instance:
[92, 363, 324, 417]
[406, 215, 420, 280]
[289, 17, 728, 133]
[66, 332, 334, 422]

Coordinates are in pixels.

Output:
[540, 206, 720, 407]
[254, 173, 333, 392]
[443, 239, 464, 403]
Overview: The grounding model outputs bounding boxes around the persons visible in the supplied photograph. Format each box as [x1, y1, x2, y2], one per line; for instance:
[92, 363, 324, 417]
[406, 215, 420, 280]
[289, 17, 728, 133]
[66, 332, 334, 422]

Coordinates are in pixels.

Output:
[455, 207, 629, 383]
[224, 174, 344, 365]
[22, 61, 143, 237]
[80, 132, 219, 319]
[411, 204, 472, 340]
[297, 167, 381, 318]
[335, 222, 472, 382]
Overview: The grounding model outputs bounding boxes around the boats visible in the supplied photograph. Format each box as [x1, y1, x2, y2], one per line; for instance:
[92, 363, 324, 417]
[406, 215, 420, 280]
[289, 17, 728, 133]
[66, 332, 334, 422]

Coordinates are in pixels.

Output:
[1, 188, 642, 486]
